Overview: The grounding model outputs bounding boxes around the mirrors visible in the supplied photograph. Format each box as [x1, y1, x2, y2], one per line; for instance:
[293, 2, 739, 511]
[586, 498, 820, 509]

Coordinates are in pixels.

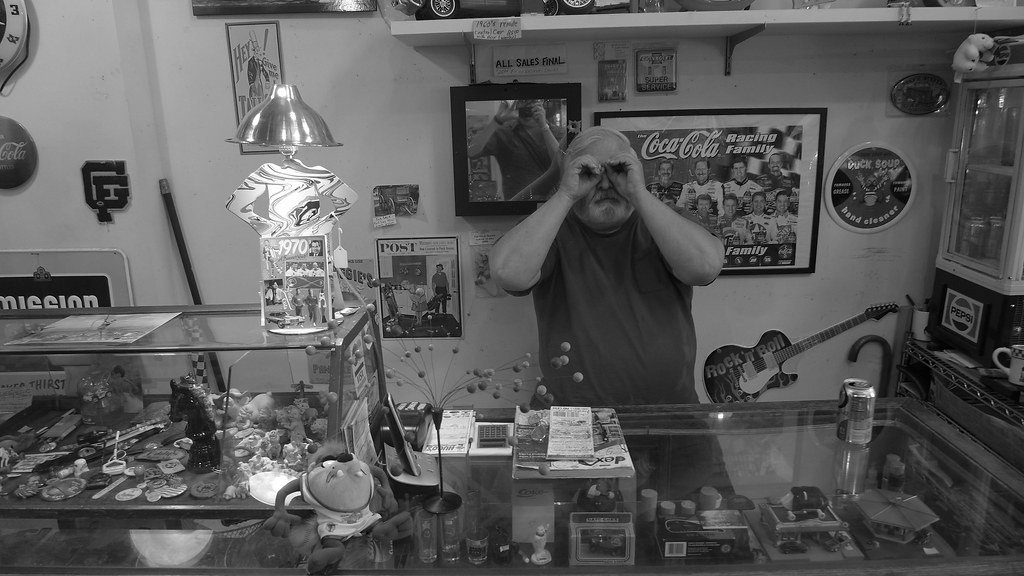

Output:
[450, 82, 582, 218]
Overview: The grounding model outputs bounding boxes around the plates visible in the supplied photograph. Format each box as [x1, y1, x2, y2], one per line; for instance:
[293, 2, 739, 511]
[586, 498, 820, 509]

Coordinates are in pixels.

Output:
[130, 530, 212, 565]
[248, 472, 301, 507]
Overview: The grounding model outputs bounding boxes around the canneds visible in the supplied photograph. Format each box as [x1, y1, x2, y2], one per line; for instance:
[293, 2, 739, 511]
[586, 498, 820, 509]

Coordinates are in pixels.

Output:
[837, 377, 876, 445]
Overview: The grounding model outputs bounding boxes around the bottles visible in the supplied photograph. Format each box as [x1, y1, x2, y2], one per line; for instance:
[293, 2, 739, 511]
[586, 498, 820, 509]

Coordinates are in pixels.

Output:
[881, 453, 905, 492]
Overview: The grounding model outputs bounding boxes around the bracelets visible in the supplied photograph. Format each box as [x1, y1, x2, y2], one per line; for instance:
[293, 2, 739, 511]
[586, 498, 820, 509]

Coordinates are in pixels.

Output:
[494, 115, 504, 126]
[541, 124, 549, 132]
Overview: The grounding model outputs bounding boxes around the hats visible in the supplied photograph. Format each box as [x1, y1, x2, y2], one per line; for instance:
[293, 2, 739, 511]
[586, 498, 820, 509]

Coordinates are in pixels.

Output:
[436, 264, 444, 270]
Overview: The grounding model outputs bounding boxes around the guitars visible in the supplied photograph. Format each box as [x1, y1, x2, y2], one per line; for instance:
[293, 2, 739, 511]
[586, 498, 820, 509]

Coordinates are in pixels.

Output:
[702, 300, 900, 404]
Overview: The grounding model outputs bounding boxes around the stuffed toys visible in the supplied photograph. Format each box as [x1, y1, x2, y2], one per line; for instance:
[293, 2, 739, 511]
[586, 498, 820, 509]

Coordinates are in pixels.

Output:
[264, 443, 413, 573]
[952, 33, 993, 71]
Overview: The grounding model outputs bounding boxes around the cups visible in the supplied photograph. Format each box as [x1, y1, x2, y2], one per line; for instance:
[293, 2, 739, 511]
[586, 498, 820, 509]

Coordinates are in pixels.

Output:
[911, 309, 931, 341]
[417, 508, 437, 563]
[465, 528, 489, 564]
[992, 345, 1024, 386]
[492, 535, 519, 565]
[441, 511, 460, 561]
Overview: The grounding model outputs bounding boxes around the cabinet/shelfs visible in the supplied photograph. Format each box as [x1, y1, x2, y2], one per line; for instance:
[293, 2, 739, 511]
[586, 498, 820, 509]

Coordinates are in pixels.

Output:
[0, 307, 1024, 576]
[936, 62, 1024, 295]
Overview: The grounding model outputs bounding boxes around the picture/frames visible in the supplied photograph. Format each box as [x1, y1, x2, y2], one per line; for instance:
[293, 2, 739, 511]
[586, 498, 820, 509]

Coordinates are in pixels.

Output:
[593, 107, 828, 274]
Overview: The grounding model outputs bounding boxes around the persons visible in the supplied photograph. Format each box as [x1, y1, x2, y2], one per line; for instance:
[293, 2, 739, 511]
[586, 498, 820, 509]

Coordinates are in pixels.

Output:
[408, 264, 450, 326]
[645, 155, 799, 246]
[489, 126, 738, 507]
[469, 99, 561, 200]
[108, 365, 142, 411]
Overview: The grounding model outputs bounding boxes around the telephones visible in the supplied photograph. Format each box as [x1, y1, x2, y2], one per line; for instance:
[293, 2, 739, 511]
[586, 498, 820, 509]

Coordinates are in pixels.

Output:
[382, 392, 441, 489]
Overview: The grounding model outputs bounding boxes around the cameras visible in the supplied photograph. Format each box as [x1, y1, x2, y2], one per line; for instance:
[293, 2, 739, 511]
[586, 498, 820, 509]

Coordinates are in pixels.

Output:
[519, 106, 534, 118]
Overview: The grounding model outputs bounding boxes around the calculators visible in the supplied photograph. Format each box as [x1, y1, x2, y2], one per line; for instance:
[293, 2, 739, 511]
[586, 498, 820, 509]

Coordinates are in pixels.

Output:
[478, 424, 509, 448]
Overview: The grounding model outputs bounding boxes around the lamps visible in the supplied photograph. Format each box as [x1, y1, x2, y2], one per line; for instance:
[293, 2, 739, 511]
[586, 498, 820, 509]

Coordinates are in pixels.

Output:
[225, 83, 344, 158]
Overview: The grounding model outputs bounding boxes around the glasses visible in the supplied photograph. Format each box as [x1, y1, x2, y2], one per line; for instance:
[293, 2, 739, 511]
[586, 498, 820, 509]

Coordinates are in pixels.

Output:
[528, 414, 546, 441]
